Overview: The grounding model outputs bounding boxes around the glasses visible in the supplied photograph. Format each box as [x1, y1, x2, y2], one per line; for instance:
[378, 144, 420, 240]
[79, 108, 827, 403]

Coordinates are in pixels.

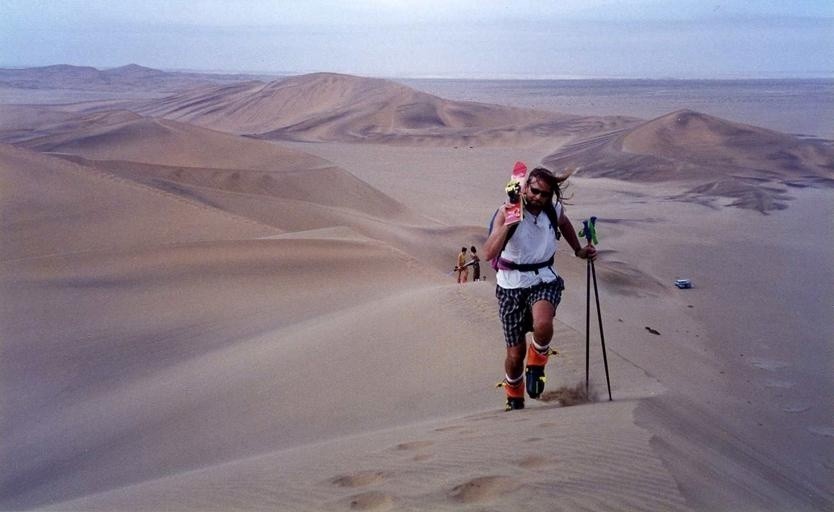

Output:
[529, 184, 550, 198]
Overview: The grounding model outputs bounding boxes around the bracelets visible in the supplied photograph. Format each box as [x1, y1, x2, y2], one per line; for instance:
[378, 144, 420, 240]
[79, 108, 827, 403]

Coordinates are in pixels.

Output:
[574, 247, 581, 256]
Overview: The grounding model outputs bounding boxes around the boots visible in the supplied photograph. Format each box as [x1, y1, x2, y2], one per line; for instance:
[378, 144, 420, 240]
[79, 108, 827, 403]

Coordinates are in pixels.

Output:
[506, 383, 525, 411]
[526, 346, 548, 396]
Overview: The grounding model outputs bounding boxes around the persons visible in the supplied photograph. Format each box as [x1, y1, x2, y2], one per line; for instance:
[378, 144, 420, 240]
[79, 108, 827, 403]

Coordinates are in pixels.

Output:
[469, 244, 481, 281]
[456, 245, 467, 285]
[482, 166, 596, 415]
